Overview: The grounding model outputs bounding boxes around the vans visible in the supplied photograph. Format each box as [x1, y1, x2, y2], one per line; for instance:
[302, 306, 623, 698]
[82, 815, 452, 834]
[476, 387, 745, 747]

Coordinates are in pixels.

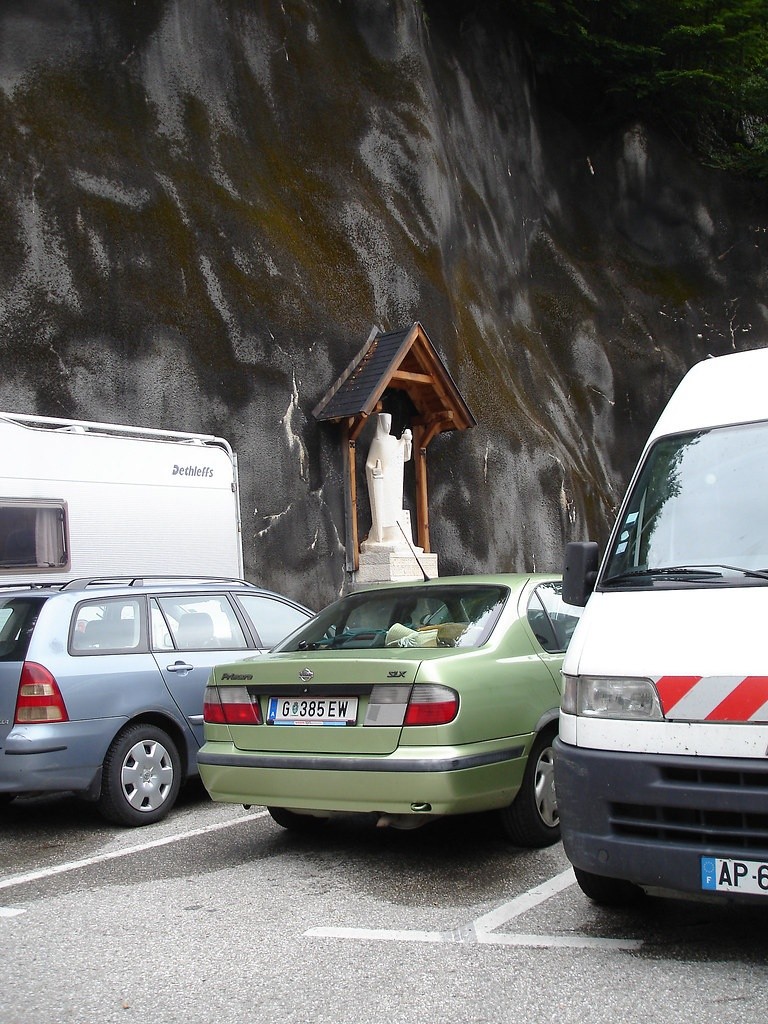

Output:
[556, 348, 768, 911]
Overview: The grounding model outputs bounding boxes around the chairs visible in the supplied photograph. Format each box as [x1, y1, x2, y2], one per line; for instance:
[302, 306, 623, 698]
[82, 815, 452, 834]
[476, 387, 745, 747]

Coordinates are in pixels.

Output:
[73, 631, 88, 650]
[114, 619, 135, 648]
[178, 612, 214, 648]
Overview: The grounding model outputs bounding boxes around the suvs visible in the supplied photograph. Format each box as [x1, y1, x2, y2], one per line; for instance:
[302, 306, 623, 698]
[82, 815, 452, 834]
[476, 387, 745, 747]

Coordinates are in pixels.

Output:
[0, 574, 353, 825]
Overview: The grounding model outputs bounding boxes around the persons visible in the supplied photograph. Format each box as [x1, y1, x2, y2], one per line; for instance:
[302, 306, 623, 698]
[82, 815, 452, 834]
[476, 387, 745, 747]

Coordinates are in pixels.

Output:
[365, 413, 412, 543]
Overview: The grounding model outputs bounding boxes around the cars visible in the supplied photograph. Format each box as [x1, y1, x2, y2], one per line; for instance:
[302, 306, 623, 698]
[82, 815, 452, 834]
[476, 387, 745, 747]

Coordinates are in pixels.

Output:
[194, 572, 585, 847]
